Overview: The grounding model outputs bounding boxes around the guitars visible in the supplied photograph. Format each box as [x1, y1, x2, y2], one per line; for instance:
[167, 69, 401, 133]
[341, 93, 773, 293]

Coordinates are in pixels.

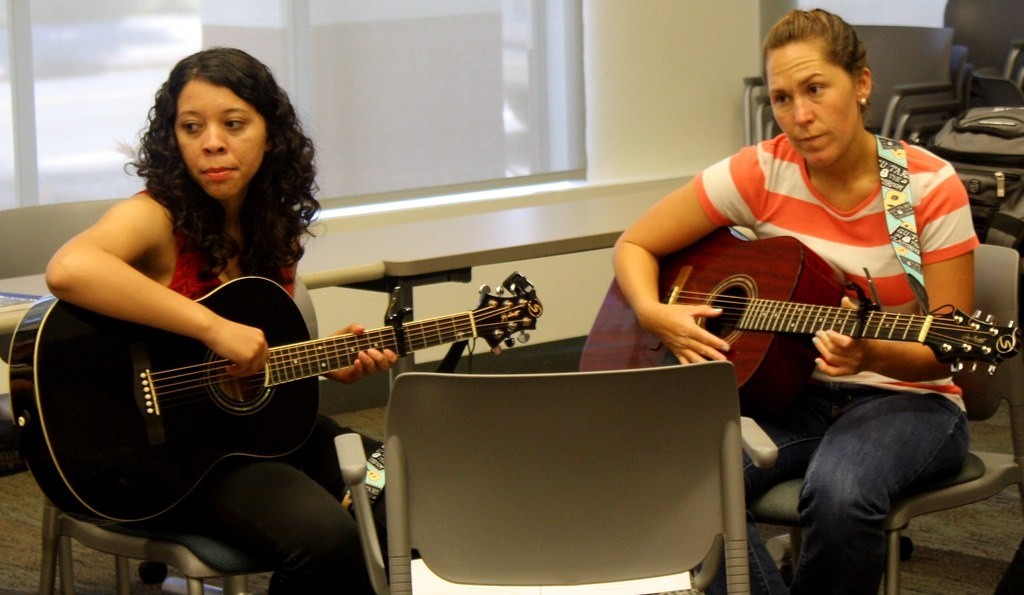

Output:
[578, 222, 1022, 424]
[8, 272, 544, 525]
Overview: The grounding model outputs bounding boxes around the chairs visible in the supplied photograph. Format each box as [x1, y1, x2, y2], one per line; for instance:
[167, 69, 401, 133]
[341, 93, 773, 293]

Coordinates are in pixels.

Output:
[333, 360, 778, 595]
[754, 243, 1024, 595]
[0, 301, 277, 595]
[742, 1, 1023, 149]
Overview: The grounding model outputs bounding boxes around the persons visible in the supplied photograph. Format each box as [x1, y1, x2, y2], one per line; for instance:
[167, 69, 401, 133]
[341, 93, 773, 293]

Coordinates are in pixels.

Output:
[612, 7, 981, 595]
[45, 46, 399, 595]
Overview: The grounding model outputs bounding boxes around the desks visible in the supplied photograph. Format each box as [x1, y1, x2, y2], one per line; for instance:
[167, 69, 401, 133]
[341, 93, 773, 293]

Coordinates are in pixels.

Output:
[0, 175, 703, 584]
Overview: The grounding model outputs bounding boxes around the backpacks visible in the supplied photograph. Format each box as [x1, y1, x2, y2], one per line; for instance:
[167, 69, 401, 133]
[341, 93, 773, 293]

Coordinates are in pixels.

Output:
[928, 105, 1024, 250]
